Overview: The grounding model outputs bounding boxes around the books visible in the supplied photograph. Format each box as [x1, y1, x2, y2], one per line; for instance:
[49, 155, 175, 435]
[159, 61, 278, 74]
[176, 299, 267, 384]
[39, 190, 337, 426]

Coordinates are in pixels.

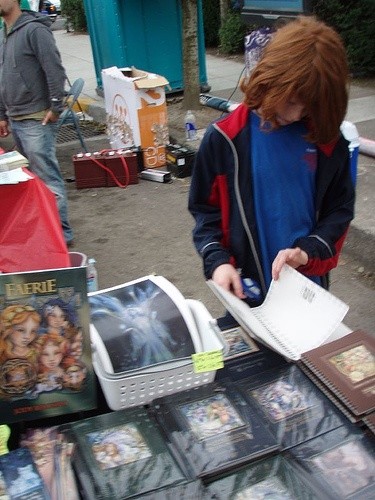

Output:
[0, 150, 30, 173]
[0, 261, 375, 500]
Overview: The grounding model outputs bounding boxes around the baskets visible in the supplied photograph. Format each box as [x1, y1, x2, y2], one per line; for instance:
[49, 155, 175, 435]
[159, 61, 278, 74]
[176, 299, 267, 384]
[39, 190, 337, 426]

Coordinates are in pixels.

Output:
[91, 298, 226, 412]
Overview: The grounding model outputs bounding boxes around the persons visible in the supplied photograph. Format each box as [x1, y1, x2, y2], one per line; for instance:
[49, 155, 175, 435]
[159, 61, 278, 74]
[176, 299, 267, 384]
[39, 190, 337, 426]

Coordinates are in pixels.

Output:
[0, 0, 77, 249]
[188, 17, 360, 315]
